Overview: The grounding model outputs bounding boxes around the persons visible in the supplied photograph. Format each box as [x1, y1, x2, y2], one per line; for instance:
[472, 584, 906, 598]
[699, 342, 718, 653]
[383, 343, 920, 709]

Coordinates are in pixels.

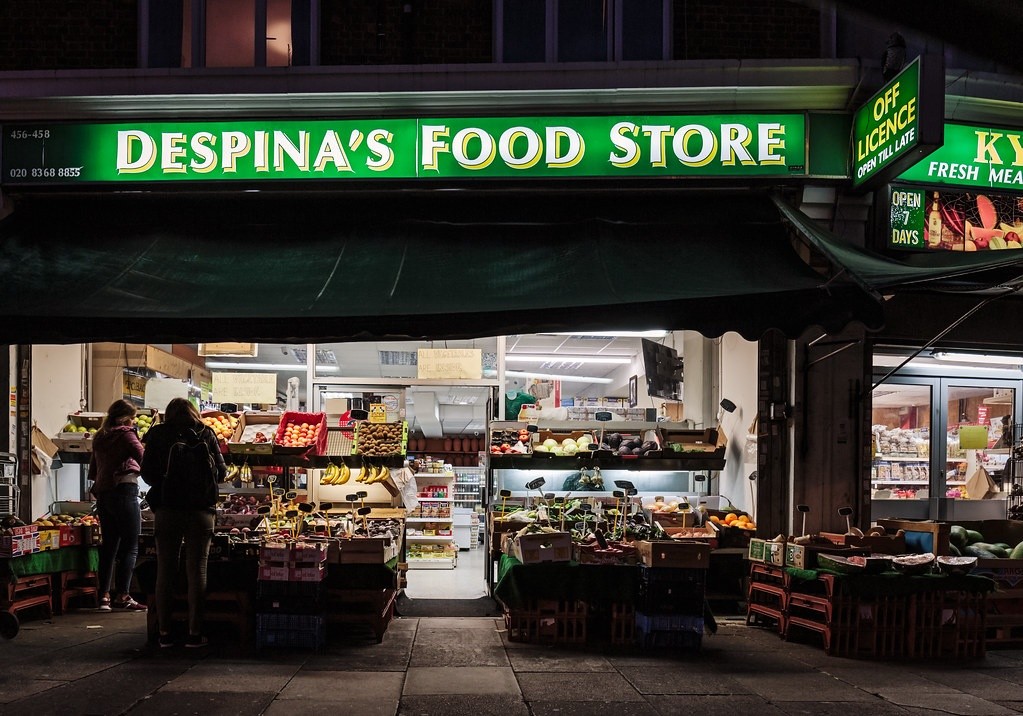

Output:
[141, 398, 227, 648]
[1001, 415, 1012, 447]
[92, 399, 149, 611]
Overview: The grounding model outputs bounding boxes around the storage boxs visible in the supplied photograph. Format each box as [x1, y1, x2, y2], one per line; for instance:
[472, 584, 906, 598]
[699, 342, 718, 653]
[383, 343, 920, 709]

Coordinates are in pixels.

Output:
[0, 409, 906, 574]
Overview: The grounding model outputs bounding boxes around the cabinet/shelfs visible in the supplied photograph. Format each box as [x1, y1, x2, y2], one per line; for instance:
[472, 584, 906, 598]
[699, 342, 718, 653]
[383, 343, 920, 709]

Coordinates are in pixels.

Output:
[983, 393, 1013, 455]
[870, 455, 1013, 500]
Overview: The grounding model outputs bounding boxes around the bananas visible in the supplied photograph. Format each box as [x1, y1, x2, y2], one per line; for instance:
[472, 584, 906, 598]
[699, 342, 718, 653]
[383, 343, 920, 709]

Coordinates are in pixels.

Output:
[222, 461, 252, 482]
[320, 462, 390, 486]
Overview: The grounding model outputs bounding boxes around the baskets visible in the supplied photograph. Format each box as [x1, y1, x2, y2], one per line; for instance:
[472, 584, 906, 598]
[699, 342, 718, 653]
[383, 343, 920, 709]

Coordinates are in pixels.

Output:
[634, 609, 706, 636]
[252, 578, 328, 615]
[502, 595, 588, 647]
[255, 612, 329, 652]
[586, 594, 636, 648]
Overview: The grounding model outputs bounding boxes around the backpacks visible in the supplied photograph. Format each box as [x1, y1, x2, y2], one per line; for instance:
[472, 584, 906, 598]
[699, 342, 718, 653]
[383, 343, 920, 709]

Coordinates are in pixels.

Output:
[157, 423, 219, 515]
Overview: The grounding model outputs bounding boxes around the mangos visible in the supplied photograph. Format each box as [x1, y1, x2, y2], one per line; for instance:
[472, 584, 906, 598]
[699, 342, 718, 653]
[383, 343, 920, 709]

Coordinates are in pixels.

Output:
[31, 511, 99, 526]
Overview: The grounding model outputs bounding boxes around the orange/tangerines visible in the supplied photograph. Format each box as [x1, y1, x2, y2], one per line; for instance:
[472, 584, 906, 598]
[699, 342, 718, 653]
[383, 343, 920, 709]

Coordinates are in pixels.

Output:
[710, 513, 754, 529]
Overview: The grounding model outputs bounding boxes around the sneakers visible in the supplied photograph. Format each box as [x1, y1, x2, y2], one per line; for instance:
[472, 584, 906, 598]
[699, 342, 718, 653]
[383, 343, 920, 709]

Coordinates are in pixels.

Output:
[98, 595, 112, 611]
[112, 595, 148, 613]
[185, 633, 210, 648]
[158, 634, 175, 648]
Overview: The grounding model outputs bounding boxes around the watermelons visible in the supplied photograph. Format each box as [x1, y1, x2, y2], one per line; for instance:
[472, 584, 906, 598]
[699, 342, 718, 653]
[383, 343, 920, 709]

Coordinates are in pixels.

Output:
[950, 525, 1023, 559]
[818, 553, 978, 572]
[942, 193, 1023, 250]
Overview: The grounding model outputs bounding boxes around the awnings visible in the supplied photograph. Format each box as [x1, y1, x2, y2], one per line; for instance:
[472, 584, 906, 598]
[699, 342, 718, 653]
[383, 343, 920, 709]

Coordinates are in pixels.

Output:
[0, 201, 856, 345]
[770, 195, 1023, 308]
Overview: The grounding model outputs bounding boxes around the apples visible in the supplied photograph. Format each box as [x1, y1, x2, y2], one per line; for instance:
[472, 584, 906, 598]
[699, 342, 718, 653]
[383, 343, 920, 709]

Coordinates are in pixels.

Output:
[252, 432, 267, 443]
[280, 422, 321, 447]
[202, 416, 238, 441]
[491, 429, 529, 454]
[65, 424, 97, 434]
[133, 415, 152, 439]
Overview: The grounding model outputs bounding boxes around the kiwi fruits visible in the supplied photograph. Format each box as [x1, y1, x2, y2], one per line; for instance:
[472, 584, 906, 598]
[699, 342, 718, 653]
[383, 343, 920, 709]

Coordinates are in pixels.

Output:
[358, 423, 403, 455]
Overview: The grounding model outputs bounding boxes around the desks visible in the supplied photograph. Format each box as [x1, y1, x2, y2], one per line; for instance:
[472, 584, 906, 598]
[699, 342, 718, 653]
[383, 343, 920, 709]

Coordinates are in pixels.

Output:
[0, 545, 1005, 660]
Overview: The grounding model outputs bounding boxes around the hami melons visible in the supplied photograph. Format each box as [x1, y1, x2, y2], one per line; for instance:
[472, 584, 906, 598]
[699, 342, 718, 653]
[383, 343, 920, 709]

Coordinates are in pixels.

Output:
[535, 437, 591, 455]
